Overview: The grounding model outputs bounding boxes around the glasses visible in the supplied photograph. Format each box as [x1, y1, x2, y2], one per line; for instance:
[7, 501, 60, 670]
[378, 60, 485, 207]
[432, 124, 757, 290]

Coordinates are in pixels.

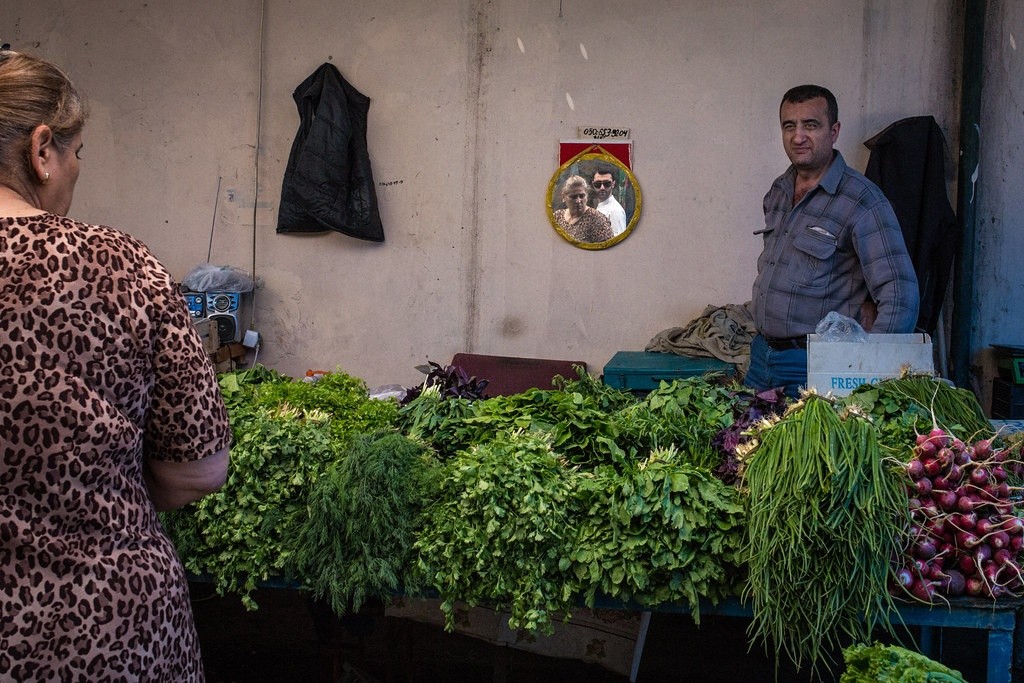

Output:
[593, 180, 612, 188]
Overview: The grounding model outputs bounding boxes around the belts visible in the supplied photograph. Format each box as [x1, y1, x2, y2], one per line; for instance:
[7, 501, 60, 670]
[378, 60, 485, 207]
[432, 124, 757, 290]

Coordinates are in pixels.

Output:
[766, 334, 807, 350]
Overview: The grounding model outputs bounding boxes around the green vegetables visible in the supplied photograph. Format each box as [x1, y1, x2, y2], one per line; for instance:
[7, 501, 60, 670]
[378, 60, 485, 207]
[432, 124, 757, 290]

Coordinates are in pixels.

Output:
[157, 362, 803, 648]
[839, 383, 931, 470]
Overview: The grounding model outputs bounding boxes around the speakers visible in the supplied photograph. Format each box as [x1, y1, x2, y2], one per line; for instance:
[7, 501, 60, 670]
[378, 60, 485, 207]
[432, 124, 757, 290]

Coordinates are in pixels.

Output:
[204, 292, 242, 343]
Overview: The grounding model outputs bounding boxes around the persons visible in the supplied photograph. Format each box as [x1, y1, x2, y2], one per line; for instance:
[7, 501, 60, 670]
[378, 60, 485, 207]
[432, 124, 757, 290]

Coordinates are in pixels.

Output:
[0, 44, 229, 683]
[744, 84, 920, 402]
[551, 165, 626, 242]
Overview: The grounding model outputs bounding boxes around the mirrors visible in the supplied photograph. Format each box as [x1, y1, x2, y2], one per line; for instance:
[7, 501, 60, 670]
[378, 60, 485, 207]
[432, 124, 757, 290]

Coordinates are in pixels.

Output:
[546, 145, 642, 251]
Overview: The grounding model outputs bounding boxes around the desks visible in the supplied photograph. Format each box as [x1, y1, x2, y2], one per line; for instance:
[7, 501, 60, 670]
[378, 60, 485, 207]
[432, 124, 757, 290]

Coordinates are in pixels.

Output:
[184, 416, 1023, 682]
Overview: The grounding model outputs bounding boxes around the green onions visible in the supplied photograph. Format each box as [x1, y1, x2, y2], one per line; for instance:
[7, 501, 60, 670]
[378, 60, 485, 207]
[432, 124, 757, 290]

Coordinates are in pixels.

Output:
[880, 366, 997, 444]
[729, 387, 936, 683]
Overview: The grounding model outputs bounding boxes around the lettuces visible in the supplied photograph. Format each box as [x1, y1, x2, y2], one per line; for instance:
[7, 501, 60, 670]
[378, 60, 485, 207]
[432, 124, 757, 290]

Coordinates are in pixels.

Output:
[836, 643, 968, 683]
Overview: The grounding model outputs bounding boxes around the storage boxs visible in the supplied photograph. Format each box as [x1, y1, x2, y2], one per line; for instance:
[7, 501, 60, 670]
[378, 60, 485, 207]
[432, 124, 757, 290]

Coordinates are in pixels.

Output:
[603, 351, 737, 401]
[991, 376, 1024, 420]
[806, 332, 935, 396]
